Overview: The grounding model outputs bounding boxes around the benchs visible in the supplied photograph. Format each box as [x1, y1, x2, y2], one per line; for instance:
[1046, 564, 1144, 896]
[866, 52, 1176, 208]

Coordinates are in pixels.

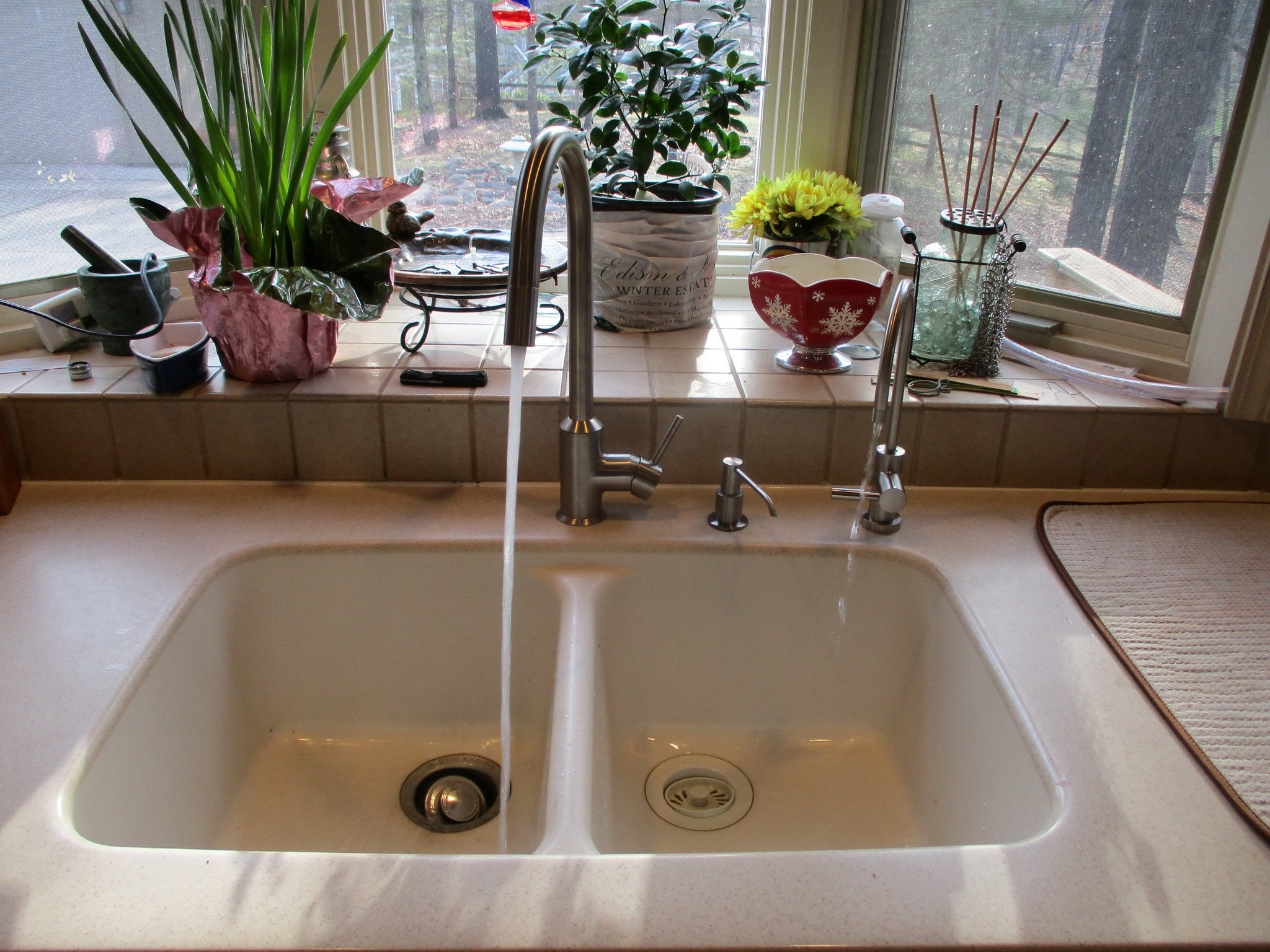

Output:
[1034, 247, 1184, 319]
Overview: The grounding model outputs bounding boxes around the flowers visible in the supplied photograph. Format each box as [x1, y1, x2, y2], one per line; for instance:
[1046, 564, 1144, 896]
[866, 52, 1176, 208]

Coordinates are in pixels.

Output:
[726, 167, 874, 256]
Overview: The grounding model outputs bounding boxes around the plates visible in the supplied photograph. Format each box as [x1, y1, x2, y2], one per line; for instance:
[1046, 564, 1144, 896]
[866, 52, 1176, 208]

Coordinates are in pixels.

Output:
[384, 229, 568, 292]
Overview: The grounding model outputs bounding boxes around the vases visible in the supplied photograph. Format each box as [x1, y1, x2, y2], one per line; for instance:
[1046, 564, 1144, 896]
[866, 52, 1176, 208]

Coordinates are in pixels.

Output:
[750, 233, 830, 262]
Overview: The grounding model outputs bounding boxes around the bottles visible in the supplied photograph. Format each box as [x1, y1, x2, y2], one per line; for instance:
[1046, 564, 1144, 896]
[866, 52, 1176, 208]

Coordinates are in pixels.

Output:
[912, 207, 1006, 362]
[854, 193, 906, 284]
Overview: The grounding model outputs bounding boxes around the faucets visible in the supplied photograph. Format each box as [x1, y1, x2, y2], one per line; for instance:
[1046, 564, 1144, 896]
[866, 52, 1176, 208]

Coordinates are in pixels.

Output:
[500, 123, 690, 531]
[829, 276, 922, 538]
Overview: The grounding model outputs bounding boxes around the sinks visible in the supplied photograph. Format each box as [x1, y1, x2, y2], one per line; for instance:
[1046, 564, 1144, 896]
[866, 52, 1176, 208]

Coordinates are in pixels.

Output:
[536, 531, 1071, 866]
[61, 539, 608, 862]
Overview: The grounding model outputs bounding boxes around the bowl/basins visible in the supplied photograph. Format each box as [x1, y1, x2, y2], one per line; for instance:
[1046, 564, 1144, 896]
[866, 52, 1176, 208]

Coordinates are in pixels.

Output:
[130, 321, 211, 391]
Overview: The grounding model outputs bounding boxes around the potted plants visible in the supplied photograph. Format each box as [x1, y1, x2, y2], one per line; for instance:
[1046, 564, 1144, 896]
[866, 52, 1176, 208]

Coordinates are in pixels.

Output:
[77, 0, 421, 380]
[522, 0, 771, 332]
